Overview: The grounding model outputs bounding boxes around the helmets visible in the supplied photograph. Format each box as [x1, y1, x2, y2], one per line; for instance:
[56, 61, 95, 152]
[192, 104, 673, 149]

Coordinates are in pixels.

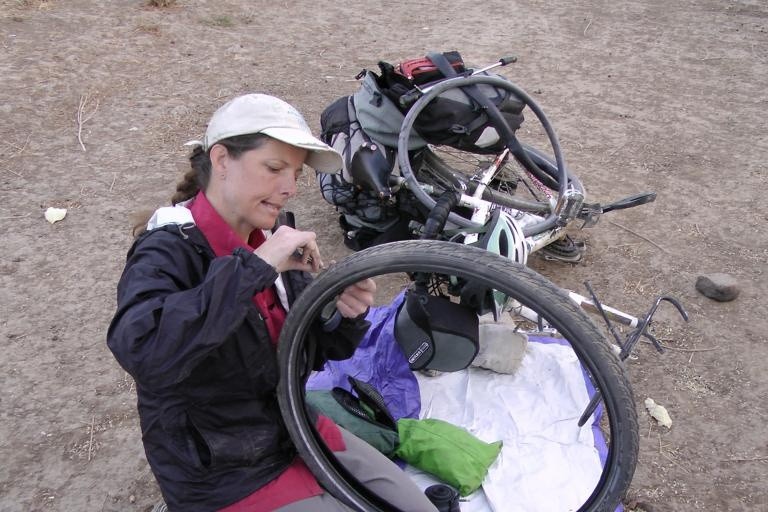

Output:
[458, 208, 529, 323]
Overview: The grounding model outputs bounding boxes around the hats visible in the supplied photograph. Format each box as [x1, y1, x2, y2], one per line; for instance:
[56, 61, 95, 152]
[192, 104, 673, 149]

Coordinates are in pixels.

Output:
[201, 93, 343, 175]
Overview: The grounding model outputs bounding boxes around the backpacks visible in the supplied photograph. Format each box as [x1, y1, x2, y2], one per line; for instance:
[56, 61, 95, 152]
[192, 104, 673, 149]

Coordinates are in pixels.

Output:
[348, 49, 529, 156]
[315, 95, 430, 252]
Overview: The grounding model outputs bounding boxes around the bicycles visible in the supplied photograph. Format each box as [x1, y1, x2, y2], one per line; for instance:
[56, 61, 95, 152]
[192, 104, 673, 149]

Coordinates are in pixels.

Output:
[275, 56, 690, 512]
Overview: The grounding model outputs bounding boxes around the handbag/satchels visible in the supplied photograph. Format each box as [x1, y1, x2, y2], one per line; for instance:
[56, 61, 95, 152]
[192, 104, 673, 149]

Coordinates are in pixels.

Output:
[305, 375, 401, 457]
[393, 290, 482, 374]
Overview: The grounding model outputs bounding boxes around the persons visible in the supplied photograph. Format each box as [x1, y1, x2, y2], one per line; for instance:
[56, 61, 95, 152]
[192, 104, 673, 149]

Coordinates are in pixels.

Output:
[104, 92, 378, 511]
